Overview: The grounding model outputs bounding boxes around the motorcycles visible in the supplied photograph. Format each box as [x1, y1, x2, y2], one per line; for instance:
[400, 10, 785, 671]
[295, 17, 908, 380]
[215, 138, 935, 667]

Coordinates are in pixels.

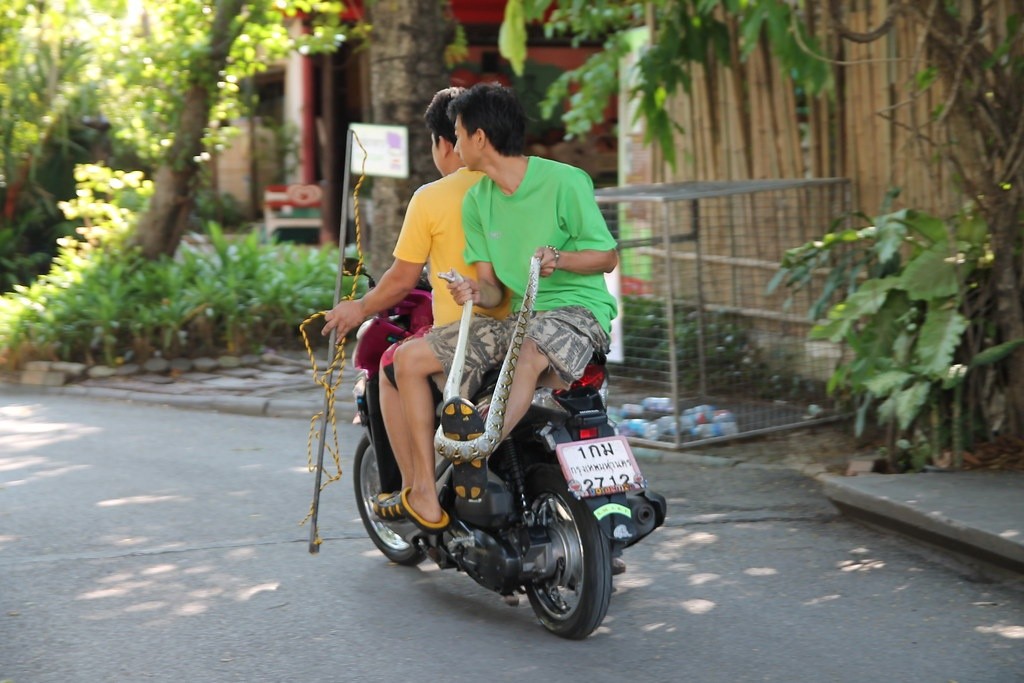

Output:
[341, 256, 667, 642]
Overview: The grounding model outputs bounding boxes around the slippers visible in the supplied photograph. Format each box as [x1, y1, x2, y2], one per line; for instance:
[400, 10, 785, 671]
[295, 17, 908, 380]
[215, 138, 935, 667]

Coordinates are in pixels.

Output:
[370, 486, 450, 535]
[441, 396, 489, 503]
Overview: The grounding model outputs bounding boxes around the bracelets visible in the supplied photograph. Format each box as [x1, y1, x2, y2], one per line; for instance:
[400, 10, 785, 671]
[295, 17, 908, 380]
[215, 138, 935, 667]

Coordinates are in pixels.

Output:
[546, 244, 560, 272]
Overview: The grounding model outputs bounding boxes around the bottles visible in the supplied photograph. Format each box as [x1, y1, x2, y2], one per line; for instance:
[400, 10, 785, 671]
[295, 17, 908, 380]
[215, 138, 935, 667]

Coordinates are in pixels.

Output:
[597, 379, 738, 442]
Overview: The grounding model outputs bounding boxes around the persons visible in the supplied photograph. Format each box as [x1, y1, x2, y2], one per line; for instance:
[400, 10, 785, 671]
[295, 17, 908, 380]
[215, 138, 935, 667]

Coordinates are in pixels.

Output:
[323, 85, 487, 489]
[373, 84, 623, 533]
